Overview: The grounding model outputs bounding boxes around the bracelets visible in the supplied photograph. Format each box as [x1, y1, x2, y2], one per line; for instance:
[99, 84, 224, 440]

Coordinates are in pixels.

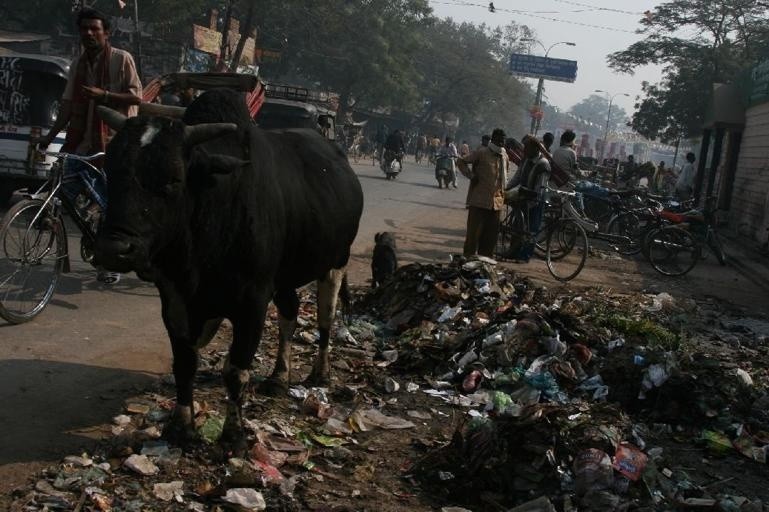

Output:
[103, 89, 110, 104]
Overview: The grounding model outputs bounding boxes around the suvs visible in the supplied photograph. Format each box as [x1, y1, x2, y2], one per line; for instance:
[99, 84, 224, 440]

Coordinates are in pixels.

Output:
[255, 84, 336, 144]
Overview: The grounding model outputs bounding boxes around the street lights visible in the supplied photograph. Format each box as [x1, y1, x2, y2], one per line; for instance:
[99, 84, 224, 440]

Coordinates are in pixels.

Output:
[594, 88, 630, 163]
[520, 34, 577, 140]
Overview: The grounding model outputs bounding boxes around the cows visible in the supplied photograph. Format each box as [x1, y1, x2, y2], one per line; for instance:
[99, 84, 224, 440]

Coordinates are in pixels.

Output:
[80, 87, 366, 459]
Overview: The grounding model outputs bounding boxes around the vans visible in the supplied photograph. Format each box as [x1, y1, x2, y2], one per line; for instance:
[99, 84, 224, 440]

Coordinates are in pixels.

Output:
[1, 55, 72, 182]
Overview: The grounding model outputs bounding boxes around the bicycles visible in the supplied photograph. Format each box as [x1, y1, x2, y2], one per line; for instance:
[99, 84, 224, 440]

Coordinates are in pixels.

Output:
[414, 147, 438, 168]
[498, 167, 726, 282]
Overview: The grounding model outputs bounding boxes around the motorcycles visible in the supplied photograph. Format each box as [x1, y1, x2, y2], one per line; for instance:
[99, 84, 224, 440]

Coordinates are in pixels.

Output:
[434, 152, 460, 190]
[383, 146, 405, 180]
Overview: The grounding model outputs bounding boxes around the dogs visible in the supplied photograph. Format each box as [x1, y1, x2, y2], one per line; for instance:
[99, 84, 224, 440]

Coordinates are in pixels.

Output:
[369, 231, 400, 288]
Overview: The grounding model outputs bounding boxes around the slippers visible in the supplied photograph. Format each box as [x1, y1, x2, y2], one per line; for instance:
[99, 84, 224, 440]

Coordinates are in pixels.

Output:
[104, 271, 121, 286]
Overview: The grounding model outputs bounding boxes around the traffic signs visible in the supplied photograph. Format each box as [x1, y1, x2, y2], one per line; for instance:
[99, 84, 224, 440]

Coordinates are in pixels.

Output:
[507, 52, 577, 86]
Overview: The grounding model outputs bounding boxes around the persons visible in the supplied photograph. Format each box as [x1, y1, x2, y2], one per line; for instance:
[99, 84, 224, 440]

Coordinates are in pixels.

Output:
[375, 128, 469, 164]
[28, 6, 144, 286]
[476, 134, 491, 150]
[676, 152, 697, 201]
[505, 134, 555, 263]
[552, 129, 607, 198]
[542, 132, 555, 152]
[456, 128, 509, 258]
[437, 136, 458, 189]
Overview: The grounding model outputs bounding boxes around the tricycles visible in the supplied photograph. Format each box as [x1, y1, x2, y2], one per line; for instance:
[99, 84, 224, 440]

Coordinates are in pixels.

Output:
[1, 73, 266, 324]
[334, 120, 376, 166]
